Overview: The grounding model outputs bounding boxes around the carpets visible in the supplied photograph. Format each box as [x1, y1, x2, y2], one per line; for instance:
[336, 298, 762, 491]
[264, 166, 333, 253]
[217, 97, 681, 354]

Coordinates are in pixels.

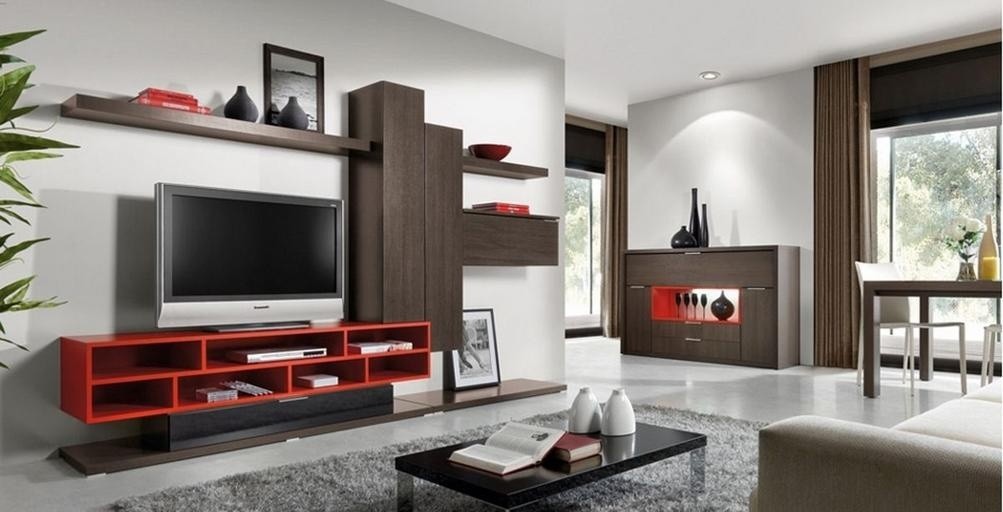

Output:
[113, 404, 772, 512]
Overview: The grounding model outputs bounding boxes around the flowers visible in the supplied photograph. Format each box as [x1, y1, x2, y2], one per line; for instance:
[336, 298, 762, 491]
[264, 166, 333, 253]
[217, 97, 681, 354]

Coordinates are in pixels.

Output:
[933, 211, 989, 262]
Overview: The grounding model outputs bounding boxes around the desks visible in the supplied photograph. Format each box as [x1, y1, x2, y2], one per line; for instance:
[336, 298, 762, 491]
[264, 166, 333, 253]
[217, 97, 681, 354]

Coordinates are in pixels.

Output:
[861, 278, 1002, 401]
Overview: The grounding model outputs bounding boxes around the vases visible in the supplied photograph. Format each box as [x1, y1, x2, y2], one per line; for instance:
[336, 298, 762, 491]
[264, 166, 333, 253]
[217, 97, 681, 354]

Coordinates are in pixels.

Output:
[670, 224, 699, 248]
[955, 261, 977, 280]
[689, 186, 703, 248]
[223, 84, 259, 123]
[701, 202, 709, 248]
[979, 214, 998, 279]
[709, 290, 735, 322]
[277, 95, 309, 130]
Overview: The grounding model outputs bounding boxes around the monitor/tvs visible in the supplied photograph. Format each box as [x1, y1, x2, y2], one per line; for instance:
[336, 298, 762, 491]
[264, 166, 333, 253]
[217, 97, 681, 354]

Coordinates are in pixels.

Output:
[156, 182, 346, 332]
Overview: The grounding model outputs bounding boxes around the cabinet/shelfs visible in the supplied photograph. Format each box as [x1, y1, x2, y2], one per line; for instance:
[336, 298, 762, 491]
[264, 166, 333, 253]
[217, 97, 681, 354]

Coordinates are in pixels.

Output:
[618, 243, 801, 371]
[58, 320, 433, 428]
[460, 207, 560, 268]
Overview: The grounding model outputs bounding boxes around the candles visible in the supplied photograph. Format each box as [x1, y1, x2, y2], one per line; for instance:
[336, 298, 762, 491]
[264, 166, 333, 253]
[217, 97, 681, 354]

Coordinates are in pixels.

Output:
[982, 255, 999, 279]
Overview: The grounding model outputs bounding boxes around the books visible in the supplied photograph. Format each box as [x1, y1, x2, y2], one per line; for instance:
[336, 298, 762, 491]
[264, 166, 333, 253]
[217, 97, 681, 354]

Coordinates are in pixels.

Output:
[451, 421, 565, 474]
[129, 88, 212, 115]
[555, 433, 602, 464]
[474, 202, 528, 215]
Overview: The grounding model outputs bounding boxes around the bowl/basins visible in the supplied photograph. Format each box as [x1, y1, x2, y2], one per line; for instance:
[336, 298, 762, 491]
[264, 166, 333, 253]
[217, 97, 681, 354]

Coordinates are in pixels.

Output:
[468, 142, 512, 161]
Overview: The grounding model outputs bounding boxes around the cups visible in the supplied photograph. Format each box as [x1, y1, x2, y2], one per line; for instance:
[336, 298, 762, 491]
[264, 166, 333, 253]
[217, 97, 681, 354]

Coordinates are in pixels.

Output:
[682, 292, 689, 323]
[981, 255, 998, 283]
[567, 386, 602, 434]
[675, 292, 682, 322]
[601, 389, 636, 436]
[691, 293, 699, 324]
[701, 293, 708, 324]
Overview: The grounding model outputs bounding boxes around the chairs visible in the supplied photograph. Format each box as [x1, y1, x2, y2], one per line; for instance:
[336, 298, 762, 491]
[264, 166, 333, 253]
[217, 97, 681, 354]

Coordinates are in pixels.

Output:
[979, 324, 1001, 391]
[856, 259, 969, 397]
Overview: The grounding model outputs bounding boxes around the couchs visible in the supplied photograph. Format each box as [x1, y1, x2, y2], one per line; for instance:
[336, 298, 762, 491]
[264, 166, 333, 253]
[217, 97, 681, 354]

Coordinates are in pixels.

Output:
[747, 374, 1001, 512]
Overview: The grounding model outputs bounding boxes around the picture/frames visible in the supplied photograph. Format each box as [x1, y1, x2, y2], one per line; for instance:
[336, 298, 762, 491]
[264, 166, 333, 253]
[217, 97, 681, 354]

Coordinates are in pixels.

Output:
[262, 41, 325, 134]
[449, 307, 503, 393]
